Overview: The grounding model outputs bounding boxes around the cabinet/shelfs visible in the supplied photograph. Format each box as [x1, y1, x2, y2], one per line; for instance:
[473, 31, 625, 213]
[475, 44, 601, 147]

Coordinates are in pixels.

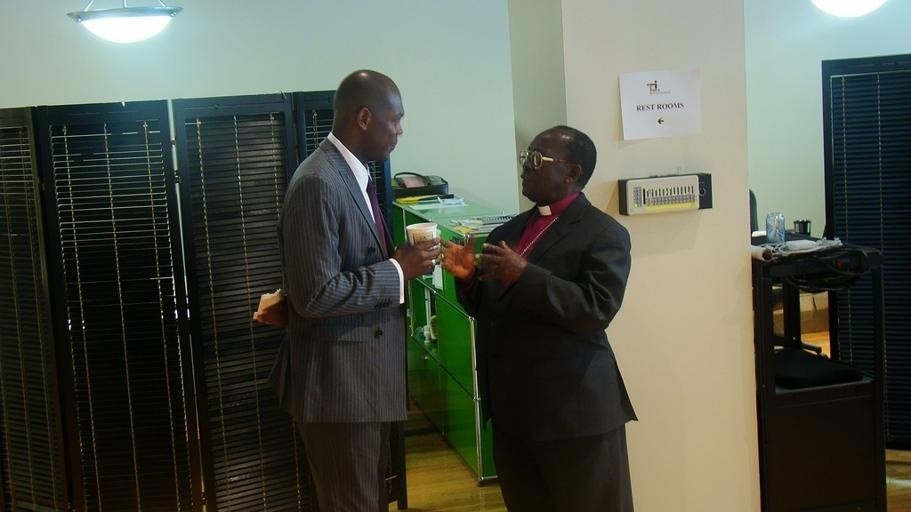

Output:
[393, 195, 509, 487]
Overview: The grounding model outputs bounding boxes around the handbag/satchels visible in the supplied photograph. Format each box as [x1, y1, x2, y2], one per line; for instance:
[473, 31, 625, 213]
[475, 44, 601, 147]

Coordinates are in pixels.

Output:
[390, 171, 449, 199]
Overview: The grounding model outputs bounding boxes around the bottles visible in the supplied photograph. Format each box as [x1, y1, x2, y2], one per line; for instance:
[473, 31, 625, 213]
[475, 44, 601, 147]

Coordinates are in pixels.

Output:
[765, 212, 785, 245]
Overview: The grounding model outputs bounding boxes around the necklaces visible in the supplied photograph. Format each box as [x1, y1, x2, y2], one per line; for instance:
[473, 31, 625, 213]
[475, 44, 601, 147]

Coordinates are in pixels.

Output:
[518, 211, 559, 257]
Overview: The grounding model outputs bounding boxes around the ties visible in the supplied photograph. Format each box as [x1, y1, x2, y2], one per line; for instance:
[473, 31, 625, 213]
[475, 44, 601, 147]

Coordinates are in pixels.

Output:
[366, 174, 389, 260]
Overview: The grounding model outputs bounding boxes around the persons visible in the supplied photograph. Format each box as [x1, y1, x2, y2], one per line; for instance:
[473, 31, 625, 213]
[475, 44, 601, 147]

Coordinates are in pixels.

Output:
[442, 125, 644, 510]
[250, 71, 445, 510]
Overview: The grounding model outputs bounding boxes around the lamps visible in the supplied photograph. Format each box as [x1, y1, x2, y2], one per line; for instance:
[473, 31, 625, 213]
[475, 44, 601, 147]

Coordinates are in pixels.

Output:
[66, 0, 184, 47]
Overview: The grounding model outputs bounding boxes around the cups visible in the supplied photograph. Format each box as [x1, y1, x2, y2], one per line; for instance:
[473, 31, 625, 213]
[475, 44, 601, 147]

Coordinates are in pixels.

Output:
[405, 223, 440, 251]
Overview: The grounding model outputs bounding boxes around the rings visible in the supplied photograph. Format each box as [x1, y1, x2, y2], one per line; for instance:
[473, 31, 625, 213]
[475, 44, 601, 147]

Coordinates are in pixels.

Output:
[432, 258, 437, 267]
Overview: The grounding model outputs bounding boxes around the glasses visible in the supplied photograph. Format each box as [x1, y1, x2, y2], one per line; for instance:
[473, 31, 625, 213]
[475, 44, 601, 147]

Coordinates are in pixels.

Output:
[518, 150, 569, 169]
